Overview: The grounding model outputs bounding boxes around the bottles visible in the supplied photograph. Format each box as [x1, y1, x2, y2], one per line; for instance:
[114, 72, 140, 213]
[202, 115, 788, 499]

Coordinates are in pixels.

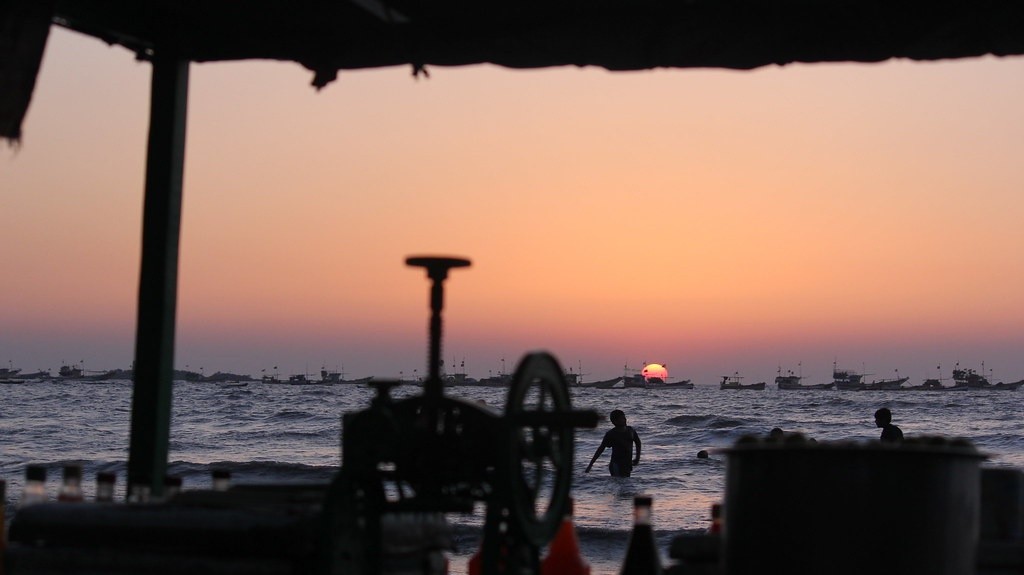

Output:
[128, 475, 152, 507]
[165, 476, 183, 501]
[58, 466, 82, 502]
[705, 504, 721, 536]
[96, 472, 116, 503]
[619, 497, 664, 575]
[212, 470, 230, 491]
[0, 479, 7, 574]
[21, 465, 47, 508]
[541, 499, 586, 575]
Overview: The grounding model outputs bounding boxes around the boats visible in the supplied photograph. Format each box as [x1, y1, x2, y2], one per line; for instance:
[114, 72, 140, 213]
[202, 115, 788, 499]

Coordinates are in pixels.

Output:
[832, 359, 910, 391]
[719, 369, 766, 390]
[621, 359, 695, 390]
[260, 355, 623, 389]
[182, 364, 249, 387]
[774, 360, 834, 389]
[904, 361, 1024, 391]
[0, 359, 132, 383]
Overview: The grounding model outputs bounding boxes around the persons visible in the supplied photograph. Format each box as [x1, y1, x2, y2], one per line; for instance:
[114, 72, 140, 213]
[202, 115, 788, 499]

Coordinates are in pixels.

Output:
[583, 409, 642, 478]
[873, 407, 904, 445]
